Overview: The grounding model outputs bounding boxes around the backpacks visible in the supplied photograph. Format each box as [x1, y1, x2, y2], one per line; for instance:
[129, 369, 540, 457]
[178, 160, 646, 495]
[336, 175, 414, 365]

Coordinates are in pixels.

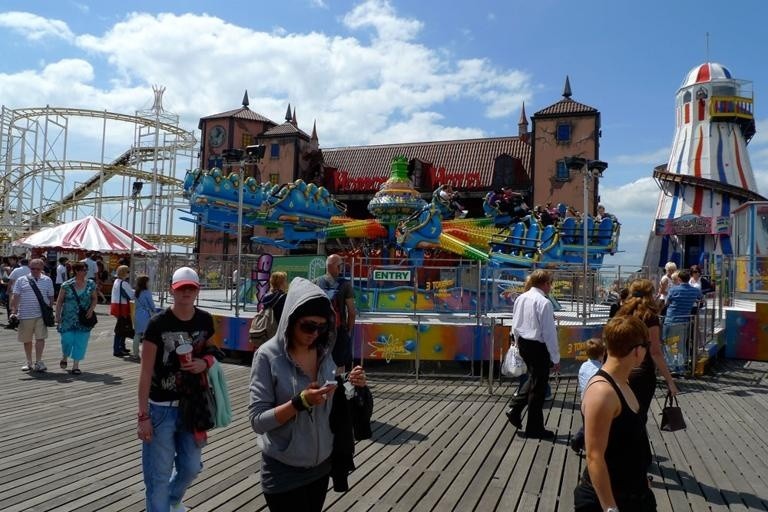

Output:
[248, 293, 285, 348]
[319, 277, 348, 334]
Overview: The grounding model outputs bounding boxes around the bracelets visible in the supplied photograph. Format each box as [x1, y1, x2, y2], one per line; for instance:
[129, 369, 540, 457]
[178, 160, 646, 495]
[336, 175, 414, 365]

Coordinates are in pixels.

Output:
[137, 413, 151, 421]
[290, 390, 315, 413]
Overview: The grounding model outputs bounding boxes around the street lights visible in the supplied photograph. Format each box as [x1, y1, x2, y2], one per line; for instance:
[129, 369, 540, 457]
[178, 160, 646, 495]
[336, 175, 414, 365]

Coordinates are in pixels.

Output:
[219, 143, 267, 315]
[131, 179, 144, 285]
[563, 154, 608, 325]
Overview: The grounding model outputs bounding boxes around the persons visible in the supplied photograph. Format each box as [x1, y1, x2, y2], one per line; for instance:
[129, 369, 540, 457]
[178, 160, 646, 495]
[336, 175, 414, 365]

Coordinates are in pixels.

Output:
[0, 250, 158, 376]
[513, 274, 554, 399]
[247, 275, 367, 512]
[136, 265, 217, 512]
[440, 183, 611, 227]
[570, 261, 716, 511]
[261, 271, 289, 326]
[504, 268, 561, 438]
[314, 253, 356, 375]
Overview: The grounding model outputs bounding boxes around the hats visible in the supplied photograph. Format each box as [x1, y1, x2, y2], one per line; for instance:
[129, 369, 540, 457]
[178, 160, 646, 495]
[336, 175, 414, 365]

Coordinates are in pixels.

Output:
[171, 267, 200, 289]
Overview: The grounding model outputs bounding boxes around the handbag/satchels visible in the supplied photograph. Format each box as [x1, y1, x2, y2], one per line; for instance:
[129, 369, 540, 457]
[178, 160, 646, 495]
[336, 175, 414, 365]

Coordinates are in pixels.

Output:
[78, 305, 97, 327]
[43, 306, 54, 327]
[661, 391, 686, 432]
[114, 316, 132, 333]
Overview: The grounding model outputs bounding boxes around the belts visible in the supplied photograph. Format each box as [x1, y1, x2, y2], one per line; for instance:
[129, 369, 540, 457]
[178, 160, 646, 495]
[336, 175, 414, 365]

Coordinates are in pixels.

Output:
[148, 397, 178, 407]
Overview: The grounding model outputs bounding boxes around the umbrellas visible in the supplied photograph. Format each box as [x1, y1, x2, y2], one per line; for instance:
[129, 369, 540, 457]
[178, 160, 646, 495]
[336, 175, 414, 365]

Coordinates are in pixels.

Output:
[11, 213, 158, 256]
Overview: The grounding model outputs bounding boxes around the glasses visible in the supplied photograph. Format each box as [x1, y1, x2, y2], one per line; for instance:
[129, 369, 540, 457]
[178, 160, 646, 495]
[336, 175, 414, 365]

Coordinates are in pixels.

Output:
[299, 318, 329, 335]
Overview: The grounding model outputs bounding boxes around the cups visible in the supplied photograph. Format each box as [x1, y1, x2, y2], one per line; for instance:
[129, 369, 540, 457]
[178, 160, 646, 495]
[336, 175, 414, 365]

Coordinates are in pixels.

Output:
[176, 344, 193, 370]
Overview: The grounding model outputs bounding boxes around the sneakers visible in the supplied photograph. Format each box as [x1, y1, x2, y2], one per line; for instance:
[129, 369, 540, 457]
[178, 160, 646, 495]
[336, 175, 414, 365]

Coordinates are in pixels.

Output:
[22, 348, 130, 375]
[505, 407, 580, 452]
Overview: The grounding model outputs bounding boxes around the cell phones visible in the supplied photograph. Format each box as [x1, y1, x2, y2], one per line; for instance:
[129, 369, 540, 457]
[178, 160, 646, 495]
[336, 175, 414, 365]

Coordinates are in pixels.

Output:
[321, 380, 338, 388]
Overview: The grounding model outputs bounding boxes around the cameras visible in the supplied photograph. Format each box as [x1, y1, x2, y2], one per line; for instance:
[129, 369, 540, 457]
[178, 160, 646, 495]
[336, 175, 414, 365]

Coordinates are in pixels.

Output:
[9, 315, 20, 328]
